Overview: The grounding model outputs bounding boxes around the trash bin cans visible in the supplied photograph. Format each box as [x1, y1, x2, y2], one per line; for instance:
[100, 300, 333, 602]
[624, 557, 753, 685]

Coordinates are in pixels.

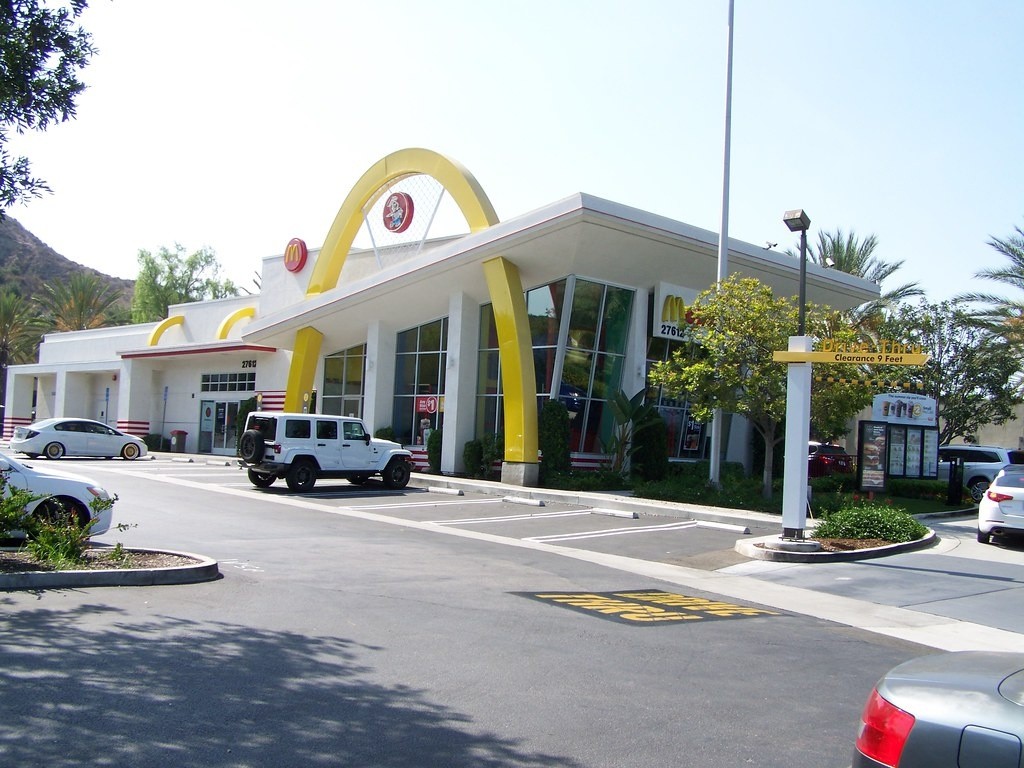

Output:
[947, 457, 964, 505]
[170, 430, 188, 453]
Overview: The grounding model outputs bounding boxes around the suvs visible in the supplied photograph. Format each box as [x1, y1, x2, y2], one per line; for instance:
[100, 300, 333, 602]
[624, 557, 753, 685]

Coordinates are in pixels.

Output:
[238, 410, 416, 494]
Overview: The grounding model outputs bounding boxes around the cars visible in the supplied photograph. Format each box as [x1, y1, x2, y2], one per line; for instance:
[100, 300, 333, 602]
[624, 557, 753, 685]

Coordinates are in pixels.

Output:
[851, 651, 1024, 768]
[8, 416, 149, 462]
[808, 441, 854, 479]
[977, 463, 1024, 545]
[0, 452, 113, 547]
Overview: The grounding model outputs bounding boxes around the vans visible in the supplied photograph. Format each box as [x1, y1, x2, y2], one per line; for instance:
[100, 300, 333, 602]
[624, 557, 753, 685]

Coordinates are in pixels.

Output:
[938, 445, 1024, 503]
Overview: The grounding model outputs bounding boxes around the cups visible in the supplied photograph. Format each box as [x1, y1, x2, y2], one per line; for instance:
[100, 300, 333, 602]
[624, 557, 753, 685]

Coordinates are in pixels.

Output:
[882, 401, 890, 416]
[902, 403, 907, 414]
[423, 429, 433, 445]
[888, 404, 895, 415]
[420, 420, 430, 444]
[895, 402, 902, 417]
[907, 403, 914, 417]
